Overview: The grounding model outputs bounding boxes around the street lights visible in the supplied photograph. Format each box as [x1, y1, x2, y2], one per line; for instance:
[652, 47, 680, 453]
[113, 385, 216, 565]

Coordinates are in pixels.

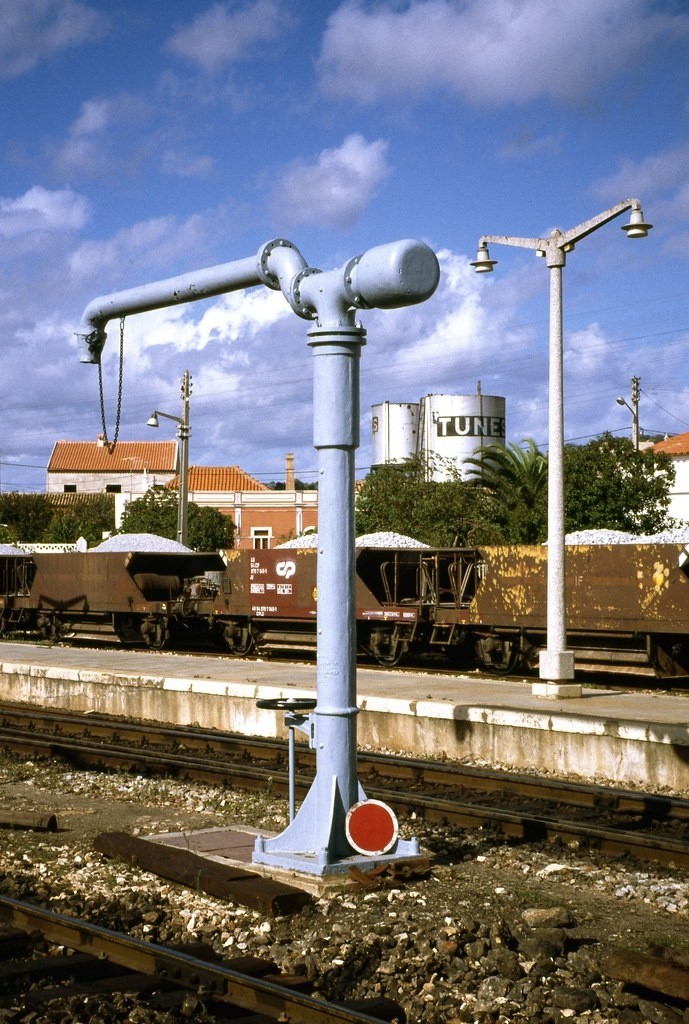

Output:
[615, 374, 642, 454]
[468, 199, 655, 700]
[146, 371, 195, 546]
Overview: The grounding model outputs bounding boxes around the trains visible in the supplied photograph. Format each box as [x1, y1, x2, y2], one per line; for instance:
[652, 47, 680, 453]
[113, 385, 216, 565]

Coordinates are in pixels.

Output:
[0, 543, 688, 691]
